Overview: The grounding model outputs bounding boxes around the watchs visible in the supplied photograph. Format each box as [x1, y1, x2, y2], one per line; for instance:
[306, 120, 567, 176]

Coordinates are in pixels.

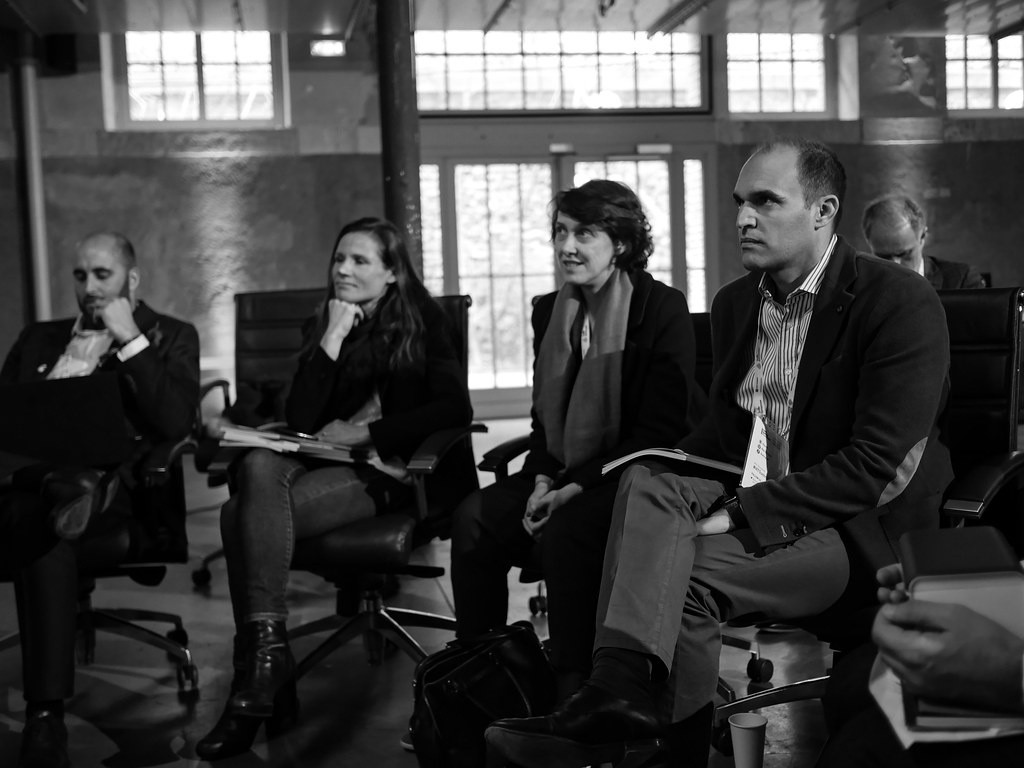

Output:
[724, 496, 746, 529]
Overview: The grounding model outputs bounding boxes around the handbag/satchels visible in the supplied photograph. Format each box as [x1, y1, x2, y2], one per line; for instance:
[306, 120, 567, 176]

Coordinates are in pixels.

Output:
[411, 619, 558, 767]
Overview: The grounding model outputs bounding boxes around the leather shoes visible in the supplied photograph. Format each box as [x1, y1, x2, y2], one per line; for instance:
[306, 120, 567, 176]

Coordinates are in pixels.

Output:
[483, 681, 665, 768]
[50, 468, 120, 539]
[19, 716, 70, 768]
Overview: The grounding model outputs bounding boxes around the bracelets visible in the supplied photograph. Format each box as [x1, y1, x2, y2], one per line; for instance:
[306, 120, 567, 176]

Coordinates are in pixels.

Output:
[534, 474, 553, 487]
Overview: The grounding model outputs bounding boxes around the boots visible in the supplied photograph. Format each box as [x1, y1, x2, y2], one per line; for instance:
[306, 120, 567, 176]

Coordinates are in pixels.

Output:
[232, 618, 300, 718]
[195, 700, 288, 758]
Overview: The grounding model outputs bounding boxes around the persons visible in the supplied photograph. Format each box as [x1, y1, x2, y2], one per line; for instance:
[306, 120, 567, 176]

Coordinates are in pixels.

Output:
[401, 138, 1024, 768]
[194, 216, 478, 768]
[0, 230, 200, 768]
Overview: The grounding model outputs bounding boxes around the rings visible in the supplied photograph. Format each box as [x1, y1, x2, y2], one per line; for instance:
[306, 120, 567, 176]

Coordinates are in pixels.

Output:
[322, 431, 326, 437]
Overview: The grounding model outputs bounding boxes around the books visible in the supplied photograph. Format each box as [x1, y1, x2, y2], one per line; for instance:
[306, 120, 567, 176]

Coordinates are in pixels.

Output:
[601, 449, 742, 475]
[216, 422, 355, 462]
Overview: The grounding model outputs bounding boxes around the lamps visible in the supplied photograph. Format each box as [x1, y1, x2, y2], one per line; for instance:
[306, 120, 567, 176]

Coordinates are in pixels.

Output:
[310, 34, 347, 58]
[545, 143, 578, 161]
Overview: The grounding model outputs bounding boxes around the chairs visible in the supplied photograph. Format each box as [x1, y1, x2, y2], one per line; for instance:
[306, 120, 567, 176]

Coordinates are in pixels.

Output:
[0, 287, 1024, 768]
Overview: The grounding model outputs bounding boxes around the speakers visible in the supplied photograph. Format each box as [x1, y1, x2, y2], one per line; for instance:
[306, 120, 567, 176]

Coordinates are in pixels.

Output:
[38, 32, 77, 78]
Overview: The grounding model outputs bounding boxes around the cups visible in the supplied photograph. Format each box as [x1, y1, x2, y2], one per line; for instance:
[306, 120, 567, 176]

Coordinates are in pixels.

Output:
[728, 712, 768, 768]
